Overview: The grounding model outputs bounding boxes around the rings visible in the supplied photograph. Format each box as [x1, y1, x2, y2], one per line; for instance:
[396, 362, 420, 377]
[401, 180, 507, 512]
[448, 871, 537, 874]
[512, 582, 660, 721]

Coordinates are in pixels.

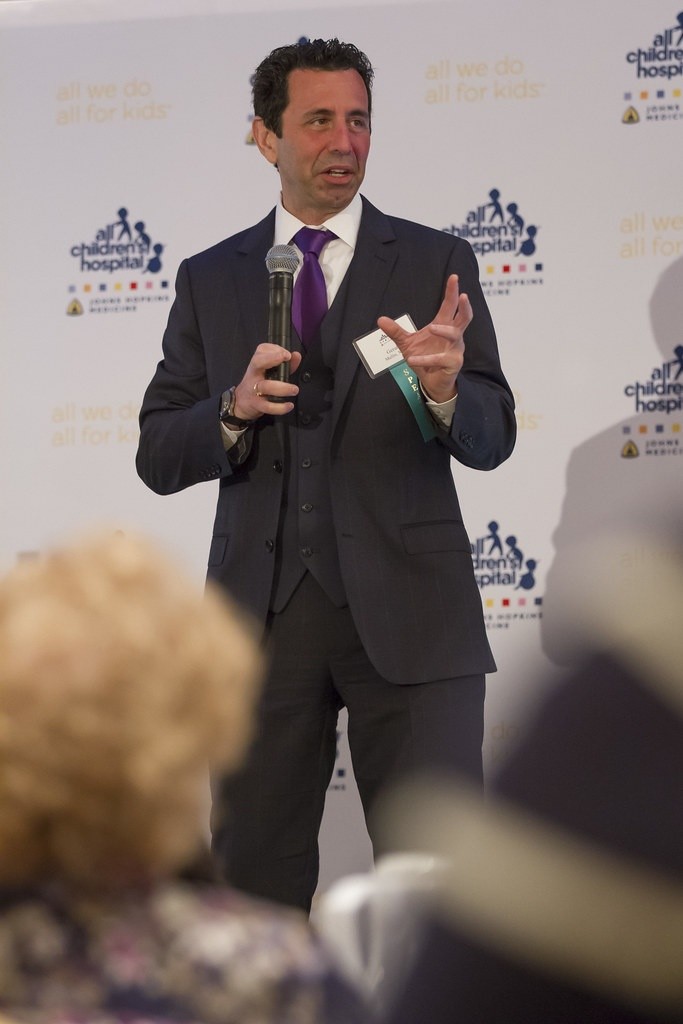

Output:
[254, 380, 267, 397]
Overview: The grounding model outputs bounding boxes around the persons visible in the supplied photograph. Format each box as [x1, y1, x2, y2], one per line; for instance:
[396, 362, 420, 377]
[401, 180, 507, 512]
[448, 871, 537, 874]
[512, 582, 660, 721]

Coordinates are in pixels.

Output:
[396, 554, 683, 1023]
[136, 38, 518, 925]
[2, 537, 378, 1023]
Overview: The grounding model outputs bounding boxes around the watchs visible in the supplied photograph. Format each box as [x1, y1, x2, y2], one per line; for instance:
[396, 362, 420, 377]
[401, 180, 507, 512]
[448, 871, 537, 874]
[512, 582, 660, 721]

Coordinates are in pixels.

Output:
[219, 385, 259, 427]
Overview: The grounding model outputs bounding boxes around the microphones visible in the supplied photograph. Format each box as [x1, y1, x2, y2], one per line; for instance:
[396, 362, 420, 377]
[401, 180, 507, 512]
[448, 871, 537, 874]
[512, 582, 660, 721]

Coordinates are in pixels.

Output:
[264, 244, 299, 402]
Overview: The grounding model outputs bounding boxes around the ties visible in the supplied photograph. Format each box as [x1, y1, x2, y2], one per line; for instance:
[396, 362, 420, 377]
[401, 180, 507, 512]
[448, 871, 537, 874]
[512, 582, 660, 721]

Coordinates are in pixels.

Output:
[290, 227, 339, 354]
